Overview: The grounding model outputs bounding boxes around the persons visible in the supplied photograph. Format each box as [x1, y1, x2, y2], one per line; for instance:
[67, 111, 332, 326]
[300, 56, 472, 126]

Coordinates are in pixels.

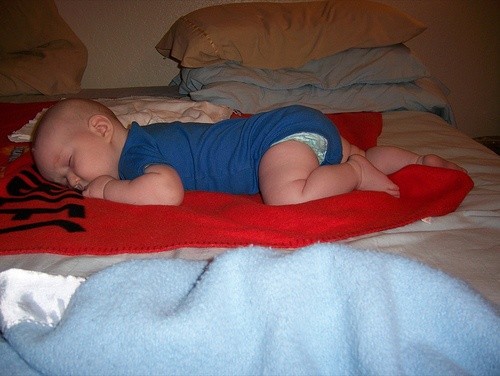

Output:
[28, 98, 472, 208]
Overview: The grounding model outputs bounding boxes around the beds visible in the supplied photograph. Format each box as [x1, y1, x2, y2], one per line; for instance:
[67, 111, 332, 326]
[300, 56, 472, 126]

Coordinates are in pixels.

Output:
[0, 86, 500, 376]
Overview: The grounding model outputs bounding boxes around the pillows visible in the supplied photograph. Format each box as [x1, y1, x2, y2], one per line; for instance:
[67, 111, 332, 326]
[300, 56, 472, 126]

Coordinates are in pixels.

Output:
[170, 42, 432, 96]
[188, 81, 457, 130]
[153, 0, 430, 69]
